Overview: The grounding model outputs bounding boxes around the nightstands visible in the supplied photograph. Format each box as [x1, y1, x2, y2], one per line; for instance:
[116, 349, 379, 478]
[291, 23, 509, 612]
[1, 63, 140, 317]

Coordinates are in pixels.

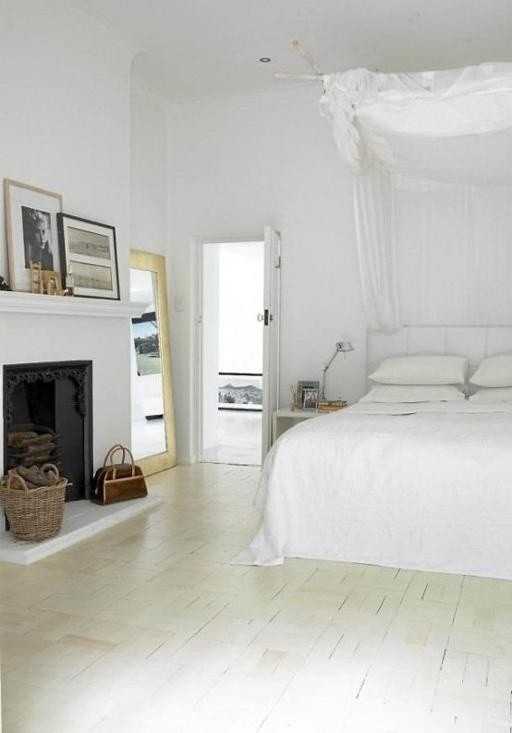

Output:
[273, 403, 328, 446]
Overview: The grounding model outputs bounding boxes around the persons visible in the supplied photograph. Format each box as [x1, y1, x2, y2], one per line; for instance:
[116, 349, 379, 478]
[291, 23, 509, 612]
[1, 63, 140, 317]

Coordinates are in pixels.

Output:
[23, 209, 53, 270]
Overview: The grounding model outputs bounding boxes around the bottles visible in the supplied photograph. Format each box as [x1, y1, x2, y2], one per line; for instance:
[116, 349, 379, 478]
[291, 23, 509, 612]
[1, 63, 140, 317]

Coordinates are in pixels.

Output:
[47, 277, 57, 295]
[65, 273, 75, 297]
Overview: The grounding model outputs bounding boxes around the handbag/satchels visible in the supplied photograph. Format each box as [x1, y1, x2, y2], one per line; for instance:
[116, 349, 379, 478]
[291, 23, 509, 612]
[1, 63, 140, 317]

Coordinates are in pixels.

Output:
[88, 443, 148, 506]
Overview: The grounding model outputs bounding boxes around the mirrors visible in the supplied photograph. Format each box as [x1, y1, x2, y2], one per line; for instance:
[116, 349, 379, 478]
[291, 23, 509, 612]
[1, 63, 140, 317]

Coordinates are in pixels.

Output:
[130, 249, 179, 478]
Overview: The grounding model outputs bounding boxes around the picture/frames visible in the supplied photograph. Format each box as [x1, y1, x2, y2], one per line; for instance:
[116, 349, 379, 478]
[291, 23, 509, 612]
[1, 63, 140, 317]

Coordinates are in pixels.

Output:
[4, 178, 62, 296]
[57, 212, 121, 302]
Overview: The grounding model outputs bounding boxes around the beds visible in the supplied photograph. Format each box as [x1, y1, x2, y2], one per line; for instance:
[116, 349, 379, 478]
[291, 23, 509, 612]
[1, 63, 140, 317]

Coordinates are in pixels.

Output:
[229, 40, 511, 583]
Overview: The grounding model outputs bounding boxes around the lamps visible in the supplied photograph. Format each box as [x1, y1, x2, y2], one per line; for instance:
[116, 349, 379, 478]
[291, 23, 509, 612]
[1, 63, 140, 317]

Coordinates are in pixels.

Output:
[320, 341, 354, 400]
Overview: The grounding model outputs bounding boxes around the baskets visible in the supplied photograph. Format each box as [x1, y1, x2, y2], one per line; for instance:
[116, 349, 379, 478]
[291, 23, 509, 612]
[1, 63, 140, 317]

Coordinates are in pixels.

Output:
[0, 462, 68, 542]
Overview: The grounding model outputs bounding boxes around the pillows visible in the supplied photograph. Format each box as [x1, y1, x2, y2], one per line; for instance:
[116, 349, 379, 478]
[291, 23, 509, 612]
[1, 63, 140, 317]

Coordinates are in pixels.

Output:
[359, 386, 466, 404]
[467, 387, 512, 405]
[366, 355, 467, 385]
[467, 357, 511, 386]
